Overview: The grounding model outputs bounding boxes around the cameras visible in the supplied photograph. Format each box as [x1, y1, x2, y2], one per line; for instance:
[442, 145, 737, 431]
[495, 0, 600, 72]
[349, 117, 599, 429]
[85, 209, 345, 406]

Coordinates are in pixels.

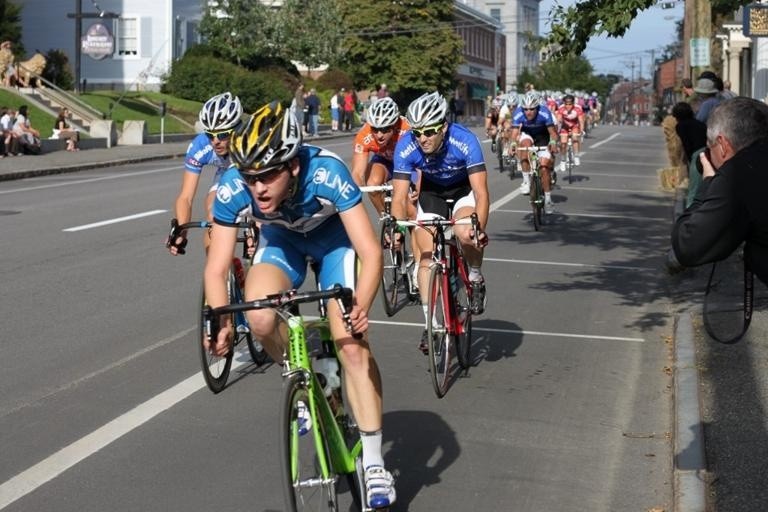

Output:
[695, 148, 718, 175]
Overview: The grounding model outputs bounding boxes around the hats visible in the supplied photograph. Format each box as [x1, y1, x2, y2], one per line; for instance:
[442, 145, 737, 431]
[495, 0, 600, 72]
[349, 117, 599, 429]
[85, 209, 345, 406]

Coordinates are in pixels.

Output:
[694, 79, 719, 94]
[680, 78, 692, 88]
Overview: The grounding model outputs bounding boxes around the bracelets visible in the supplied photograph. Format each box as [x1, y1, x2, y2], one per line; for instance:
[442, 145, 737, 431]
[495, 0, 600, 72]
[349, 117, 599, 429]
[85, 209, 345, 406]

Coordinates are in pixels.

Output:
[510, 142, 517, 147]
[548, 140, 556, 145]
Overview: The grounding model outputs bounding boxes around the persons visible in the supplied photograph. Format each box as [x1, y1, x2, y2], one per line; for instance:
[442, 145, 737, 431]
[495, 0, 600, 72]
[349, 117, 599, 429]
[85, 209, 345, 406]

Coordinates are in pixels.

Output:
[507, 92, 559, 216]
[456, 95, 466, 122]
[291, 83, 322, 137]
[554, 95, 586, 171]
[537, 88, 601, 153]
[383, 90, 488, 356]
[672, 96, 768, 288]
[350, 96, 423, 292]
[663, 72, 737, 209]
[485, 92, 522, 171]
[0, 40, 81, 159]
[200, 100, 399, 510]
[166, 89, 265, 333]
[365, 84, 391, 109]
[328, 87, 364, 132]
[449, 93, 459, 123]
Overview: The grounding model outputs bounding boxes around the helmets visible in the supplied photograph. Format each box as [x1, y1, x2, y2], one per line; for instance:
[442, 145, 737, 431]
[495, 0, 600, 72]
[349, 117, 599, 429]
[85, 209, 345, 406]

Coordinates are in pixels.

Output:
[492, 88, 596, 106]
[228, 102, 303, 171]
[199, 92, 243, 130]
[366, 97, 399, 128]
[407, 91, 448, 129]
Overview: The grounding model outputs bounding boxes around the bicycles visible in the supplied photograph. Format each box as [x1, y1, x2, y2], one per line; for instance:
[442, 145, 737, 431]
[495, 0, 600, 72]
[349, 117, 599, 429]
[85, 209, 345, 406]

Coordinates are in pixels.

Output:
[557, 132, 584, 182]
[581, 114, 589, 135]
[485, 127, 503, 173]
[163, 217, 268, 395]
[197, 284, 372, 510]
[381, 214, 486, 400]
[359, 182, 419, 315]
[511, 144, 557, 230]
[498, 126, 516, 177]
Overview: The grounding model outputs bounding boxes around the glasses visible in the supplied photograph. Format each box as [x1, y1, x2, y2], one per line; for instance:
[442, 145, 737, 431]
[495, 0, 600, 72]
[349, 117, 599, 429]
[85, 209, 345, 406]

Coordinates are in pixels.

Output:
[508, 105, 517, 107]
[239, 165, 285, 185]
[370, 126, 392, 133]
[523, 108, 535, 111]
[412, 124, 443, 137]
[205, 128, 235, 139]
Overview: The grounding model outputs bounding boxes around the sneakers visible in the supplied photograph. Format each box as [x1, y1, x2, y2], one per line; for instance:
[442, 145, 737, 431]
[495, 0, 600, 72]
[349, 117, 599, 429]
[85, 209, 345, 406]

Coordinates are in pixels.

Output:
[561, 161, 566, 171]
[492, 143, 523, 172]
[412, 263, 419, 288]
[521, 182, 531, 194]
[419, 329, 436, 350]
[543, 202, 554, 215]
[296, 400, 312, 435]
[303, 132, 320, 138]
[364, 465, 396, 508]
[383, 225, 391, 247]
[575, 157, 580, 167]
[332, 129, 353, 133]
[470, 275, 487, 315]
[234, 311, 250, 333]
